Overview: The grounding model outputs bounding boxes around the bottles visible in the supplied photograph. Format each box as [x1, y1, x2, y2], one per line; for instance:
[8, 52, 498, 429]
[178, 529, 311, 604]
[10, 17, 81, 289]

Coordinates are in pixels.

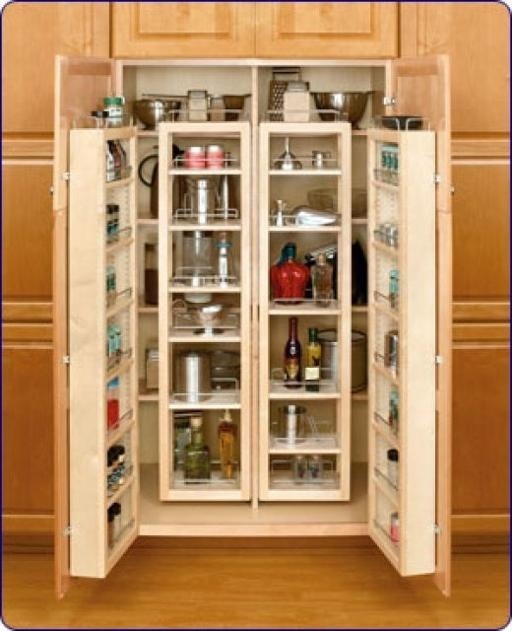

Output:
[186, 89, 210, 121]
[282, 317, 303, 389]
[270, 241, 311, 305]
[295, 454, 323, 486]
[371, 144, 399, 479]
[168, 231, 241, 288]
[172, 410, 212, 486]
[390, 513, 402, 539]
[311, 251, 335, 307]
[89, 98, 130, 129]
[211, 94, 226, 120]
[304, 326, 320, 392]
[218, 152, 236, 223]
[143, 345, 159, 392]
[150, 146, 184, 220]
[280, 82, 313, 121]
[217, 410, 242, 482]
[143, 242, 176, 308]
[107, 140, 125, 544]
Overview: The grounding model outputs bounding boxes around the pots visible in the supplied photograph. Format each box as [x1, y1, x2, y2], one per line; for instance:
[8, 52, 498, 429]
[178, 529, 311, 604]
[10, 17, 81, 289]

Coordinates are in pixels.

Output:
[134, 101, 180, 130]
[311, 89, 377, 128]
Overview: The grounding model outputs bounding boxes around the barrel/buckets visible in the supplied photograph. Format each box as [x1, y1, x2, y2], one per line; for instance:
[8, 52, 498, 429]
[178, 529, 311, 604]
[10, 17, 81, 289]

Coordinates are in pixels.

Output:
[278, 405, 307, 443]
[170, 350, 213, 402]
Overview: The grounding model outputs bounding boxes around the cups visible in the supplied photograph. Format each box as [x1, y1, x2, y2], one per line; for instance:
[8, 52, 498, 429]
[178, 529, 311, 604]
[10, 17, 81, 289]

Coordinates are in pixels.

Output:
[180, 142, 223, 169]
[185, 178, 217, 223]
[273, 197, 290, 226]
[311, 150, 330, 168]
[315, 331, 367, 396]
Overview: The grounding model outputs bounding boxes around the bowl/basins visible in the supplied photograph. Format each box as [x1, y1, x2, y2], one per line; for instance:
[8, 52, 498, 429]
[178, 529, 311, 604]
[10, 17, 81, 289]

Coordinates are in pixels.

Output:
[383, 116, 427, 130]
[186, 301, 230, 327]
[213, 349, 241, 389]
[306, 188, 368, 216]
